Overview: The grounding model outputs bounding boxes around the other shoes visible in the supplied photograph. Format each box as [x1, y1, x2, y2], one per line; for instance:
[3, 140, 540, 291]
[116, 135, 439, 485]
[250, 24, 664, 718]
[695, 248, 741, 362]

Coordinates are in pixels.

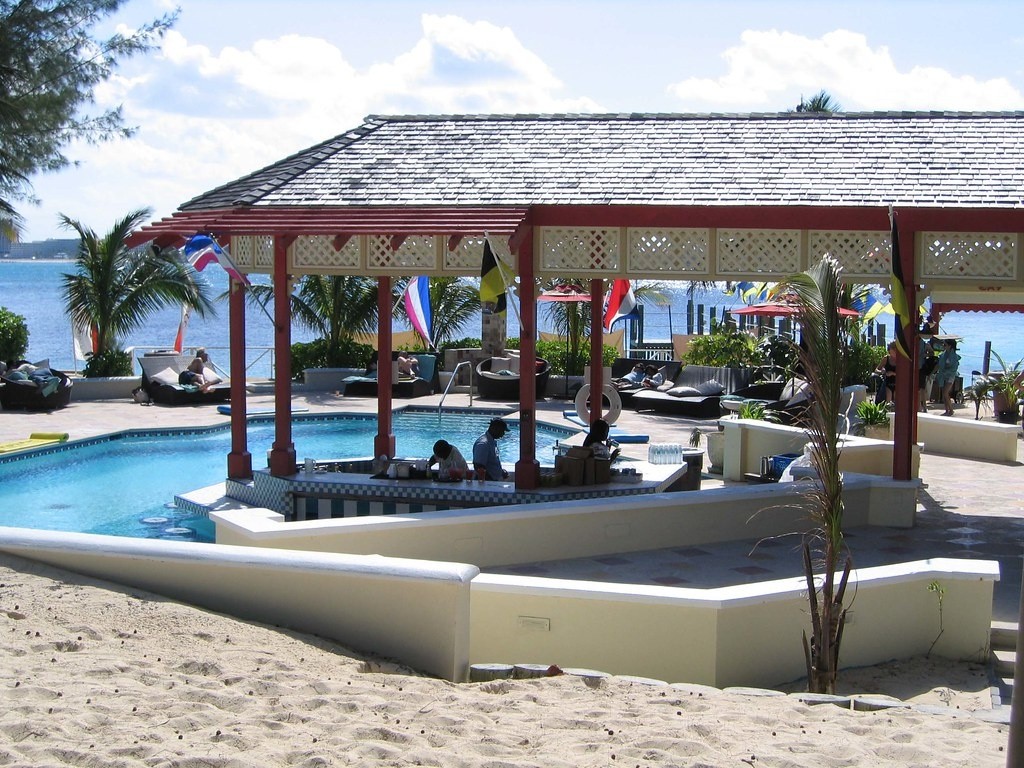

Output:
[941, 411, 955, 416]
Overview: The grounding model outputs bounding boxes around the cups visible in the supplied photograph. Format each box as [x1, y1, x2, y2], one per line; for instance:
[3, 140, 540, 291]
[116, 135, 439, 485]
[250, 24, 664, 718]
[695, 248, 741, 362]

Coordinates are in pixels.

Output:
[465, 470, 473, 483]
[478, 469, 487, 484]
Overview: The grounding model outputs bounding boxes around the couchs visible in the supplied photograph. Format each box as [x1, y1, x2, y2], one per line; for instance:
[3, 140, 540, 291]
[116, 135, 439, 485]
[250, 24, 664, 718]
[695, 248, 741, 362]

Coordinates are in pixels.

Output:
[0, 360, 73, 412]
[476, 356, 551, 400]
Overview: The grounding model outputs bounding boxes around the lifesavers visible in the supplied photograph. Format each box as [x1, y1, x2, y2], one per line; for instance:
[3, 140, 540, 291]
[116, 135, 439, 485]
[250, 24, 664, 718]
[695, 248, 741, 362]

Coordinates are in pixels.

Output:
[164, 502, 180, 508]
[575, 383, 624, 425]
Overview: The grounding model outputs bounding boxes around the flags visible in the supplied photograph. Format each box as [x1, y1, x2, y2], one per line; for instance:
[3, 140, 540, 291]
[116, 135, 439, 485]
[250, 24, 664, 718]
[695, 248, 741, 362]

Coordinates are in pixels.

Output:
[890, 211, 913, 362]
[724, 279, 781, 306]
[480, 239, 515, 321]
[174, 282, 193, 352]
[604, 278, 641, 335]
[184, 233, 251, 286]
[69, 282, 101, 360]
[846, 285, 933, 345]
[405, 276, 436, 349]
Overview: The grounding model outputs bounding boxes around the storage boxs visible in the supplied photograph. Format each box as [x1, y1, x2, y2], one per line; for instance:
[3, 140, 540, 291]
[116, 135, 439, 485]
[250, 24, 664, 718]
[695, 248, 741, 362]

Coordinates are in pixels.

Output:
[773, 452, 803, 478]
[514, 454, 540, 491]
[568, 445, 595, 486]
[555, 455, 585, 487]
[594, 456, 610, 484]
[610, 473, 643, 484]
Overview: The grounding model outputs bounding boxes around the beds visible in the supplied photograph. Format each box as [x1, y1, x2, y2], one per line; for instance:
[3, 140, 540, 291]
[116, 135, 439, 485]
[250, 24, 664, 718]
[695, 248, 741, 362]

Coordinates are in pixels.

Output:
[136, 356, 231, 406]
[569, 357, 682, 409]
[630, 363, 754, 418]
[340, 351, 439, 398]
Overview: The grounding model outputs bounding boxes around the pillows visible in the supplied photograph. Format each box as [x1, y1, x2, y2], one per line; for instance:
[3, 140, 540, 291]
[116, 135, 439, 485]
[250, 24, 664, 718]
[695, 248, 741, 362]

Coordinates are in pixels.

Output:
[696, 379, 727, 396]
[495, 370, 516, 376]
[658, 365, 668, 383]
[34, 358, 50, 369]
[786, 382, 813, 406]
[491, 356, 510, 373]
[203, 367, 222, 382]
[508, 352, 520, 374]
[149, 367, 179, 385]
[721, 394, 746, 402]
[16, 364, 39, 373]
[657, 378, 674, 392]
[536, 361, 545, 373]
[743, 399, 769, 406]
[28, 368, 54, 385]
[780, 377, 806, 399]
[667, 386, 702, 398]
[6, 372, 27, 381]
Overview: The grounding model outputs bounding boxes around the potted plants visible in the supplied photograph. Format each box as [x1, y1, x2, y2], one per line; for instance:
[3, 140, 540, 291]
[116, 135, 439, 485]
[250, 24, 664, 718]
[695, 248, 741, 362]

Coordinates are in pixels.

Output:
[963, 349, 1024, 416]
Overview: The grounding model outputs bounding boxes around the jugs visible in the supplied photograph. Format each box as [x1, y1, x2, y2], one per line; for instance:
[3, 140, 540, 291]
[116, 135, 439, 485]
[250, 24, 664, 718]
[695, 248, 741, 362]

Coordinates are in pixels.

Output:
[304, 457, 316, 473]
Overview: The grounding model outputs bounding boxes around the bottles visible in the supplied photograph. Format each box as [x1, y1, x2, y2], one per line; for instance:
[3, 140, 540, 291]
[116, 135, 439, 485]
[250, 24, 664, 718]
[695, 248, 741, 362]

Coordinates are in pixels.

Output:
[334, 463, 343, 481]
[647, 442, 683, 464]
[425, 464, 432, 480]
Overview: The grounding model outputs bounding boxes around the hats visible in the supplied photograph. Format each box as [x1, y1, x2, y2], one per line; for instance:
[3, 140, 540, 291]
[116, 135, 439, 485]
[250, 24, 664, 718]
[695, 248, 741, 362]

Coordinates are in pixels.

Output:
[490, 417, 512, 432]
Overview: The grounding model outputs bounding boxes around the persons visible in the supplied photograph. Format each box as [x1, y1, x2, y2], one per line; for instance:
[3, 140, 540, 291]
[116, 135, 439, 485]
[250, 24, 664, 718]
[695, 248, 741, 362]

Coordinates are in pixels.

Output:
[397, 350, 421, 379]
[178, 347, 215, 394]
[610, 363, 663, 390]
[875, 330, 961, 417]
[12, 360, 59, 398]
[473, 417, 511, 481]
[426, 440, 473, 479]
[583, 420, 620, 466]
